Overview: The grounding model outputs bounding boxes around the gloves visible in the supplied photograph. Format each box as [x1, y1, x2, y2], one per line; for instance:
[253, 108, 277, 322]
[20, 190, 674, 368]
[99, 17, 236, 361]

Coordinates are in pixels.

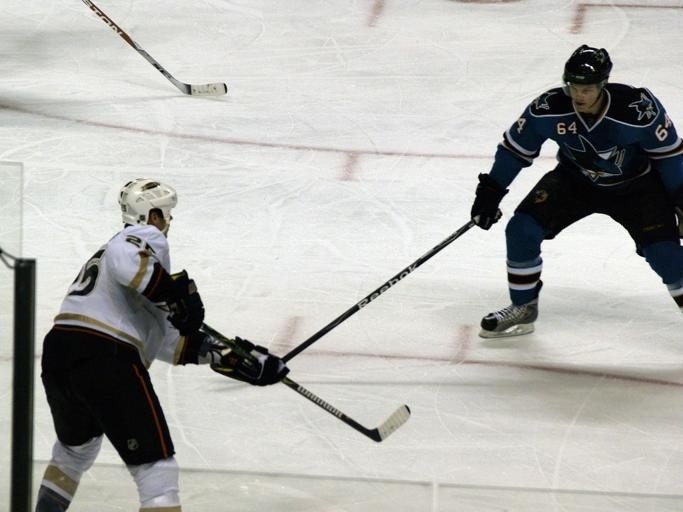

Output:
[164, 269, 205, 336]
[471, 172, 510, 230]
[210, 336, 289, 386]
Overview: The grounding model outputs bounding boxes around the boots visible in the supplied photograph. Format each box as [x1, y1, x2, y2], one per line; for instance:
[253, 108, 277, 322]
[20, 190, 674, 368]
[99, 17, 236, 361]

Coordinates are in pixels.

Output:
[480, 280, 543, 332]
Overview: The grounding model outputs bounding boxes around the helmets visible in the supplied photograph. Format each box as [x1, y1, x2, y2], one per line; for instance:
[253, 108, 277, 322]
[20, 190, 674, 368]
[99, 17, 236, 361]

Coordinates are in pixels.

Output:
[561, 44, 612, 100]
[118, 178, 177, 238]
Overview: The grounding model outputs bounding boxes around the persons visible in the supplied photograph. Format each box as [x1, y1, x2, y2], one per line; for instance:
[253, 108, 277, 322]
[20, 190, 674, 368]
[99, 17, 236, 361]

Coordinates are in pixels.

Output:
[471, 45, 683, 331]
[36, 178, 290, 512]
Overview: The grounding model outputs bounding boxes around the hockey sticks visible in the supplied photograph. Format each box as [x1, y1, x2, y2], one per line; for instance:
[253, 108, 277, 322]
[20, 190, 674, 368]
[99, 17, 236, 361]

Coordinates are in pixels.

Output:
[83, 0, 227, 96]
[199, 323, 411, 442]
